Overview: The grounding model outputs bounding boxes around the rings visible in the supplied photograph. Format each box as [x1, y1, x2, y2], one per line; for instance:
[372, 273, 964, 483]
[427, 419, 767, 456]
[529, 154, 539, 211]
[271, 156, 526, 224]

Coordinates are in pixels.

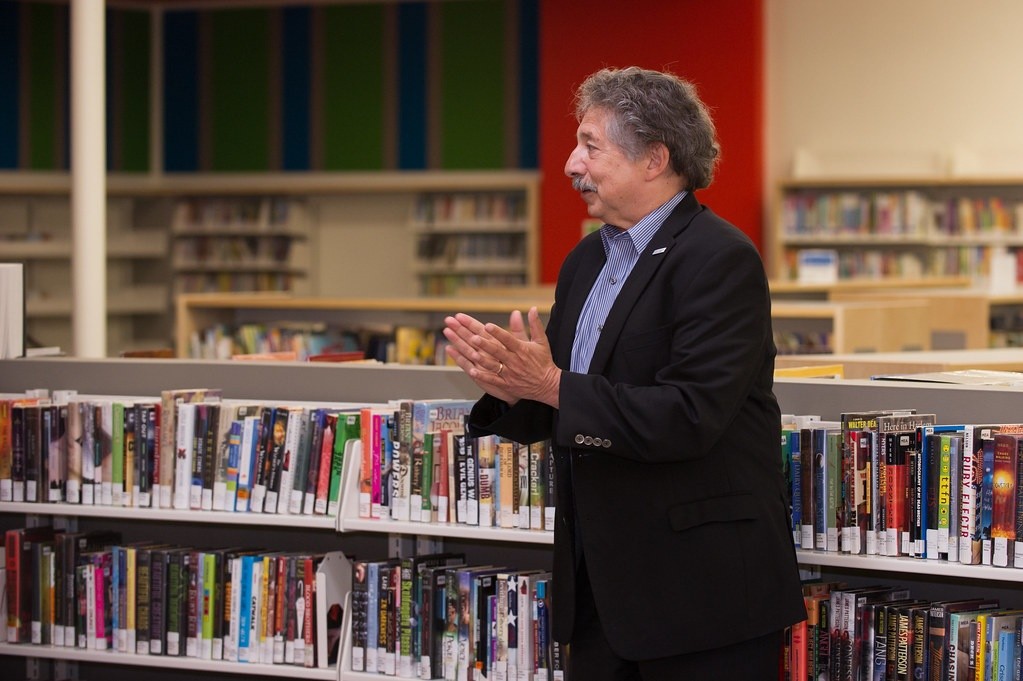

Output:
[496, 363, 503, 375]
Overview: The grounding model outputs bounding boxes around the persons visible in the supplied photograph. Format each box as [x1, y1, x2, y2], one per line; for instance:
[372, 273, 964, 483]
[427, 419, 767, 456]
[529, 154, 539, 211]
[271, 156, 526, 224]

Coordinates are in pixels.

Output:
[442, 66, 809, 681]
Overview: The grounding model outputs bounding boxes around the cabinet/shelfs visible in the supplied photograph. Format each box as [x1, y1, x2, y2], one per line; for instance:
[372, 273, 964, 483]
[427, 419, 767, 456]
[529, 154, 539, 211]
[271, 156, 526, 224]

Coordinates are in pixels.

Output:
[0, 167, 1023, 681]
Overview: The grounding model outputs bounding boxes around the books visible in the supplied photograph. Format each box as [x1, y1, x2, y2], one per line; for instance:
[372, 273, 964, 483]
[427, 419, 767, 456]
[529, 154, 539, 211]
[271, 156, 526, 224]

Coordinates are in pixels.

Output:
[180, 184, 1023, 392]
[0, 387, 1023, 681]
[0, 261, 66, 362]
